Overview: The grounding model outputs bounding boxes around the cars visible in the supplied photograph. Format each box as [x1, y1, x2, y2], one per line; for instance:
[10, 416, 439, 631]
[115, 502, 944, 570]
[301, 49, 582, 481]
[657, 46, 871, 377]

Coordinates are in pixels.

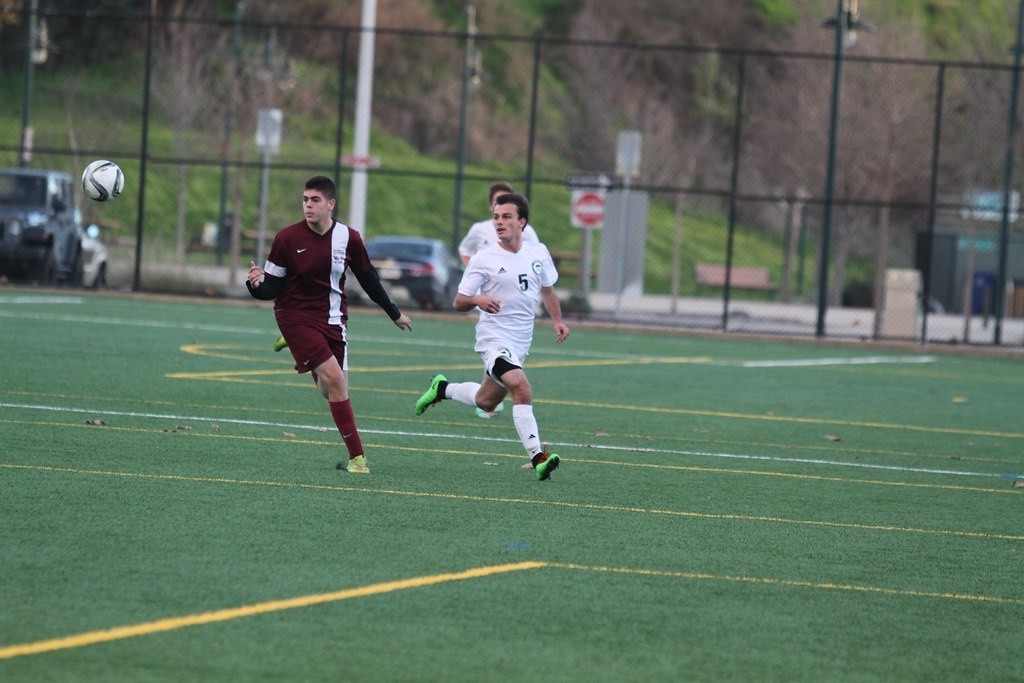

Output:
[75, 211, 109, 292]
[364, 235, 463, 310]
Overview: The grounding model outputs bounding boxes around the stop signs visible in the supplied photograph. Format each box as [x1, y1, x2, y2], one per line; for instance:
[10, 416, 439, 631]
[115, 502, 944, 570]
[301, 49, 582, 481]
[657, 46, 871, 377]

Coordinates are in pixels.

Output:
[568, 193, 604, 230]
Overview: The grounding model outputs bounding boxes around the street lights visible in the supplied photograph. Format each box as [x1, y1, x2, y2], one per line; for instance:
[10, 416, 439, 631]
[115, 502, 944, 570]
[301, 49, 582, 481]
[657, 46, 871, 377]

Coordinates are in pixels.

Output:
[815, 1, 877, 341]
[15, 1, 51, 169]
[451, 7, 484, 257]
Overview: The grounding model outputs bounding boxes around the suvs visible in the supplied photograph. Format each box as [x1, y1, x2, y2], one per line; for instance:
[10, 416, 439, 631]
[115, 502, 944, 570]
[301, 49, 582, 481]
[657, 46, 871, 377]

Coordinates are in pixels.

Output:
[0, 169, 85, 288]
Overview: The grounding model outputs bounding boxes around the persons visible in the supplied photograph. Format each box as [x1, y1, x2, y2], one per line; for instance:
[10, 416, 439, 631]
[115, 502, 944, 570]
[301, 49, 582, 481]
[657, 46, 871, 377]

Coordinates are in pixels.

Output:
[245, 175, 412, 474]
[415, 195, 570, 482]
[458, 182, 541, 419]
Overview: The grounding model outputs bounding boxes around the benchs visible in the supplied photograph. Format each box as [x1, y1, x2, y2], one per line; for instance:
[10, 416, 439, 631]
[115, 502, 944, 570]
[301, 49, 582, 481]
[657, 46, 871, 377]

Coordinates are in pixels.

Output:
[188, 230, 276, 258]
[550, 249, 595, 287]
[85, 218, 137, 255]
[694, 263, 783, 304]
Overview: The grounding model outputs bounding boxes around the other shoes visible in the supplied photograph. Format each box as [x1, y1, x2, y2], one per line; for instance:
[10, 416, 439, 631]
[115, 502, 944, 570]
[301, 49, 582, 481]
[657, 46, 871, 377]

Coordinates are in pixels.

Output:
[475, 401, 504, 419]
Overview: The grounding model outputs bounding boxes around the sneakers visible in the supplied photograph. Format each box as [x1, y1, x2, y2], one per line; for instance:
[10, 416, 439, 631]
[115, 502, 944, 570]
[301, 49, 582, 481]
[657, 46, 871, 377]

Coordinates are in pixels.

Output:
[535, 446, 560, 482]
[416, 374, 447, 415]
[348, 453, 370, 474]
[273, 334, 288, 352]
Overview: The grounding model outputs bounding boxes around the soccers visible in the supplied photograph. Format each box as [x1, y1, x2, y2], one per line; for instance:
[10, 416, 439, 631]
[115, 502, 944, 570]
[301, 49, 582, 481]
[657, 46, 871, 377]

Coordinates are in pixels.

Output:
[82, 160, 125, 202]
